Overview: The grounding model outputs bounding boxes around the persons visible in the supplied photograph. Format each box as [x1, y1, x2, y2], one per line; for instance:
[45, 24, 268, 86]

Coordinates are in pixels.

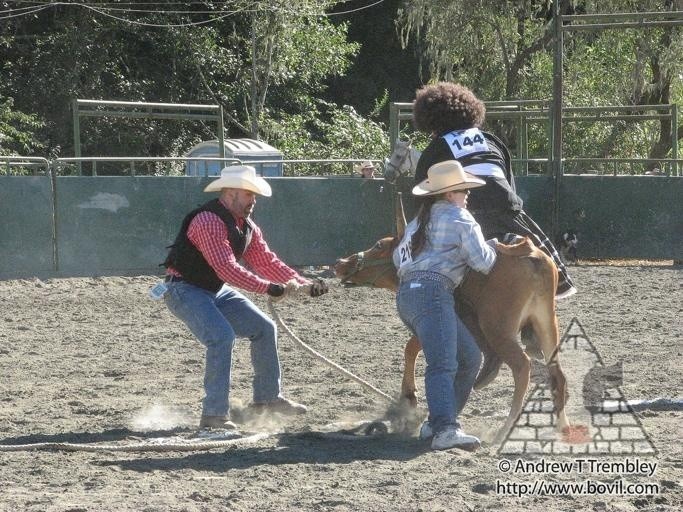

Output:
[361, 160, 375, 178]
[392, 160, 500, 450]
[163, 165, 329, 430]
[413, 80, 577, 390]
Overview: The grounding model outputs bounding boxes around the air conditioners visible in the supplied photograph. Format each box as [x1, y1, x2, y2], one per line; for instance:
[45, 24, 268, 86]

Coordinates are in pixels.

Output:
[555, 228, 578, 265]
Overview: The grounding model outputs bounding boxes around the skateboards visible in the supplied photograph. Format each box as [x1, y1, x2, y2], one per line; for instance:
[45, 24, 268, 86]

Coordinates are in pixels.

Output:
[333, 191, 572, 447]
[528, 327, 625, 444]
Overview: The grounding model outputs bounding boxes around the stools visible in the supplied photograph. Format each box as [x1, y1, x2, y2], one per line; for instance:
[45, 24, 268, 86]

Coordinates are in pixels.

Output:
[164, 274, 181, 281]
[402, 270, 455, 288]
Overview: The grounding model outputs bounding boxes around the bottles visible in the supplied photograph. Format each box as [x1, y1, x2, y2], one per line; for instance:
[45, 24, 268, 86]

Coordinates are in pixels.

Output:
[354, 160, 376, 174]
[412, 159, 486, 197]
[204, 166, 272, 198]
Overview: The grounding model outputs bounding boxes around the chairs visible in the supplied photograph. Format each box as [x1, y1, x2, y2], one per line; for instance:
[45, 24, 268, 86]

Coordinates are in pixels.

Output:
[420, 420, 433, 439]
[431, 428, 482, 451]
[254, 399, 308, 413]
[200, 414, 237, 428]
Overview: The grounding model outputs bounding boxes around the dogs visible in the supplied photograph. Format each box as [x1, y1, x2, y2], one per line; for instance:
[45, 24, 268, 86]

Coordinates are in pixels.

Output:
[383, 137, 422, 184]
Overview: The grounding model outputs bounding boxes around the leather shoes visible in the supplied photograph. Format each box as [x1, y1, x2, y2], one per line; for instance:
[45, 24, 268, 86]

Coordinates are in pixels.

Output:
[304, 278, 329, 298]
